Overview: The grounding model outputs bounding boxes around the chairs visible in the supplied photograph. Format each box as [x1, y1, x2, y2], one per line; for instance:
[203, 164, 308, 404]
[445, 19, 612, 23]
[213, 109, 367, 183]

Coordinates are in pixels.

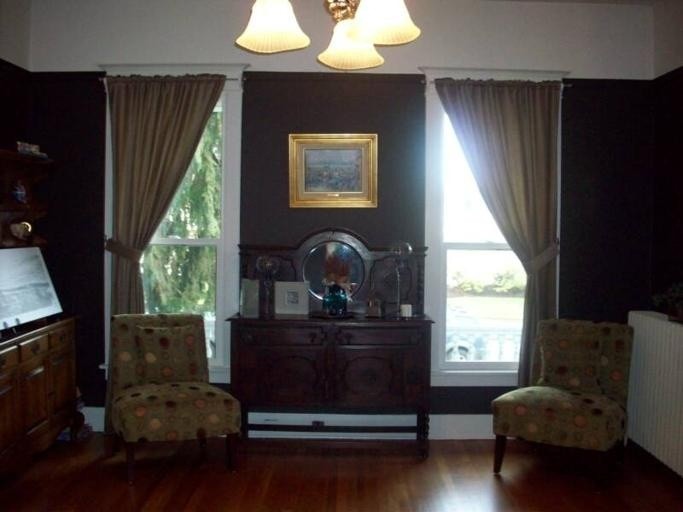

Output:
[489, 317, 635, 477]
[109, 310, 242, 471]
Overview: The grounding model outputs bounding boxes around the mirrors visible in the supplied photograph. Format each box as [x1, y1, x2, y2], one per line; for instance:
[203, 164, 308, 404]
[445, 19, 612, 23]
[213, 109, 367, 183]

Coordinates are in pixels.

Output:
[302, 240, 365, 301]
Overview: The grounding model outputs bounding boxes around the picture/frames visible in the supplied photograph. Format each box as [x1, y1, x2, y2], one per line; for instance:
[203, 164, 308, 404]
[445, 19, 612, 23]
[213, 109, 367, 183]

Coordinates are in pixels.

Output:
[288, 133, 378, 210]
[275, 281, 311, 315]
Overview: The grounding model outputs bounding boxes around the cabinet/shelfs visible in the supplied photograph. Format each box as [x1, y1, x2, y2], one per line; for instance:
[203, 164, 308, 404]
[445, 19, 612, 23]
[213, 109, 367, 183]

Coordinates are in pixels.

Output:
[0, 148, 54, 259]
[0, 316, 79, 483]
[224, 228, 434, 461]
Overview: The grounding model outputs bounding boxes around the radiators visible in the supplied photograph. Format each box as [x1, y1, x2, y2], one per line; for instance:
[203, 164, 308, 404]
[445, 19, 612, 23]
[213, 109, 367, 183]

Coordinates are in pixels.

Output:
[621, 310, 682, 483]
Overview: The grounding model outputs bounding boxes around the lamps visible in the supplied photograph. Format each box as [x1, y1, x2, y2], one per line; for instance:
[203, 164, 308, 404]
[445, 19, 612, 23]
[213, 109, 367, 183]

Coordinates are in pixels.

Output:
[236, 0, 421, 71]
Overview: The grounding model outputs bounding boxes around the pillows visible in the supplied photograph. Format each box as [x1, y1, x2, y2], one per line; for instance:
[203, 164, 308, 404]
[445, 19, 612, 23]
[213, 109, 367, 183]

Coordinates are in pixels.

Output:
[138, 324, 203, 382]
[540, 334, 603, 390]
